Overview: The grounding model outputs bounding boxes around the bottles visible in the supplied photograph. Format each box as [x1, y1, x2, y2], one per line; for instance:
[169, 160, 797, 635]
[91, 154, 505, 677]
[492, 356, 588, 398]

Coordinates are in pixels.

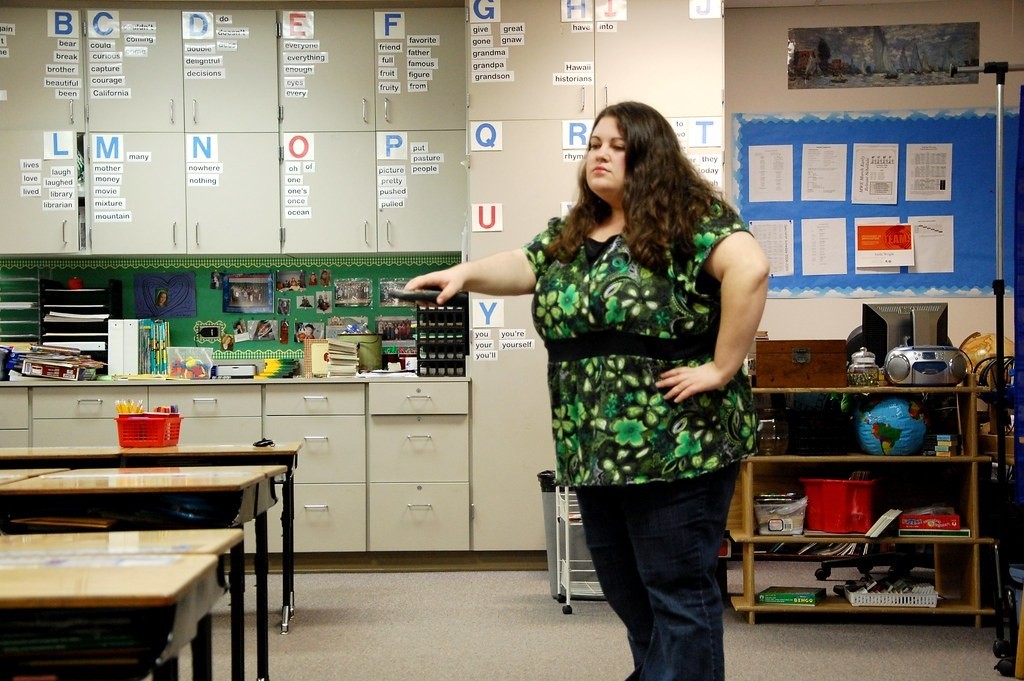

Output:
[343, 321, 371, 334]
[382, 319, 411, 340]
[757, 408, 788, 456]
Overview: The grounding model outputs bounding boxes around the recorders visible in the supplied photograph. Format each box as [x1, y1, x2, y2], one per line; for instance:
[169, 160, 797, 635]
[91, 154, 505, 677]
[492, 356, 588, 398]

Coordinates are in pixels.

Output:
[884, 346, 968, 387]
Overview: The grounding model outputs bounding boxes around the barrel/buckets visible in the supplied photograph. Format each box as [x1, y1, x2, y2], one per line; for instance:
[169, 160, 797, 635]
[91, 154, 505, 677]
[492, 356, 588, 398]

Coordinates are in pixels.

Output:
[338, 333, 382, 374]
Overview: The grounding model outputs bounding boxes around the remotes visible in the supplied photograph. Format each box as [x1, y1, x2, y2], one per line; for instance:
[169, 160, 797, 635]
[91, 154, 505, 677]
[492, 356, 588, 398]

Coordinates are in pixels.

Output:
[387, 289, 469, 307]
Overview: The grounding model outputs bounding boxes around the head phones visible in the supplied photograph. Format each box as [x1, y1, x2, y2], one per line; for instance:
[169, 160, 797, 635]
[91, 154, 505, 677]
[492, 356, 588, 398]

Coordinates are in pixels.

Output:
[971, 356, 1015, 409]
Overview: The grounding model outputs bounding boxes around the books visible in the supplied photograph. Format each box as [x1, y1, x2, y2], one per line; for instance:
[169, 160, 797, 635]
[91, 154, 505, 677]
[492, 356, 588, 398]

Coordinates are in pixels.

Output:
[327, 336, 360, 378]
[863, 508, 896, 536]
[0, 614, 140, 668]
[773, 541, 870, 556]
[0, 277, 171, 381]
[870, 508, 903, 538]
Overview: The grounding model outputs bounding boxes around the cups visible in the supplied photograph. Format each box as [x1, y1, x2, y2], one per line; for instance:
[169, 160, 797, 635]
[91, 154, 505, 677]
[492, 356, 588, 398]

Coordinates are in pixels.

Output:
[382, 354, 398, 370]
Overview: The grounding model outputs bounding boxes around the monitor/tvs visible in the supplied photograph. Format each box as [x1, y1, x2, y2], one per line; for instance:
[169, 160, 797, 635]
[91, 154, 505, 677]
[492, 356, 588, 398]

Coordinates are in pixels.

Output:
[862, 304, 948, 368]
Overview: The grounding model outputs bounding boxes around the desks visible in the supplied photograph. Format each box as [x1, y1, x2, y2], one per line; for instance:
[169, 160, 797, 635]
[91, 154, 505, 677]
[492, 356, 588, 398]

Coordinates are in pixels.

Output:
[0, 465, 288, 681]
[0, 447, 131, 469]
[979, 422, 1014, 467]
[123, 443, 305, 635]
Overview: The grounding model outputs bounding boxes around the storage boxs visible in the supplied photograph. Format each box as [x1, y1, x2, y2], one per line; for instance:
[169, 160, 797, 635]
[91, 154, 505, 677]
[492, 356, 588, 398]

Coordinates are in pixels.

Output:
[755, 340, 847, 388]
[114, 417, 183, 447]
[934, 434, 958, 457]
[758, 586, 826, 607]
[754, 504, 806, 535]
[898, 508, 971, 537]
[798, 476, 881, 534]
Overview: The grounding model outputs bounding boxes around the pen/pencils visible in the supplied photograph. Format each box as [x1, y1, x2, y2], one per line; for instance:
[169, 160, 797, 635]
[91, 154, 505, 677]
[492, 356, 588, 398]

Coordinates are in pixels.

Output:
[112, 397, 147, 415]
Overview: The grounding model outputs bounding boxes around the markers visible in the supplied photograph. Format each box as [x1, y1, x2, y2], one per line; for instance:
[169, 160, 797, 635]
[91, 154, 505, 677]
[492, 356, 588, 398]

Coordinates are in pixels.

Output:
[152, 404, 179, 414]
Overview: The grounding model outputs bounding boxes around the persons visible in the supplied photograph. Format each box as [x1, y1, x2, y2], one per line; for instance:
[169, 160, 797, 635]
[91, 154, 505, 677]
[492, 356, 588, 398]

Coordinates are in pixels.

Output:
[378, 320, 412, 340]
[398, 98, 771, 680]
[212, 269, 370, 340]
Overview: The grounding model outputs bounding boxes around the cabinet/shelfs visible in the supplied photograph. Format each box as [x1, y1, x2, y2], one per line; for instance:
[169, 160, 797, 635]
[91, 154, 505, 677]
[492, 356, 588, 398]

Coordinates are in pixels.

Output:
[730, 373, 997, 625]
[553, 480, 605, 614]
[0, 0, 726, 572]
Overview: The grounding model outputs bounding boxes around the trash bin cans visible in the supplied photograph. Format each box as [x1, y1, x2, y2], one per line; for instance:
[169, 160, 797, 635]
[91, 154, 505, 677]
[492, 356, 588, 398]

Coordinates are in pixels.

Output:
[1004, 563, 1024, 662]
[538, 470, 607, 601]
